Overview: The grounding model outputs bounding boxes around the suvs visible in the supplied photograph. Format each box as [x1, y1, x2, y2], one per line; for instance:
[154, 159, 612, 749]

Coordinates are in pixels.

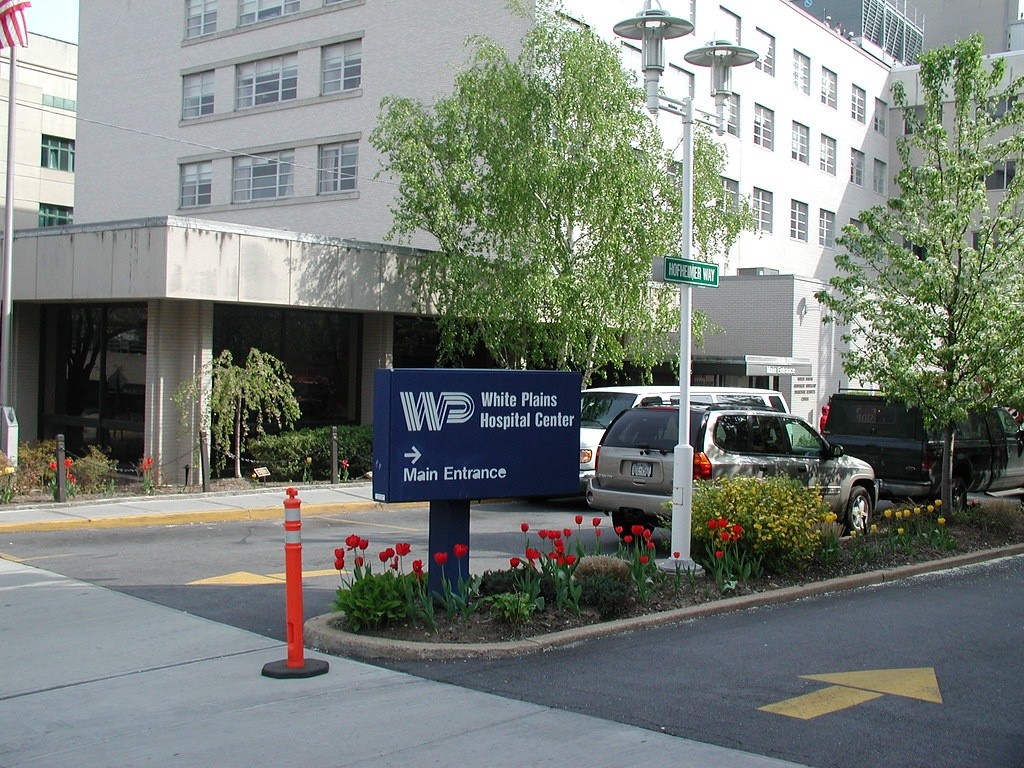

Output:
[586, 396, 880, 548]
[818, 390, 1024, 514]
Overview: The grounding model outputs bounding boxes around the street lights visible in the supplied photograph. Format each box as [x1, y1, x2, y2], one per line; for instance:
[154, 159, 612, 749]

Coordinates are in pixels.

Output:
[613, 1, 760, 580]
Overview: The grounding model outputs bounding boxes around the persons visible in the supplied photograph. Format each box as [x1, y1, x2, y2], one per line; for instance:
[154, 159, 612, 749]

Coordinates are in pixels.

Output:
[819, 406, 829, 434]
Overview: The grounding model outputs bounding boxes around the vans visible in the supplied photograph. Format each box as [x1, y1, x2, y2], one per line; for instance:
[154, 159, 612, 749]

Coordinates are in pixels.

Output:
[544, 386, 794, 501]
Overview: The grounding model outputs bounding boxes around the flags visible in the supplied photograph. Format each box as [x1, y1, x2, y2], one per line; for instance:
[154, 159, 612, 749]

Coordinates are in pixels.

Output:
[0, 0, 31, 48]
